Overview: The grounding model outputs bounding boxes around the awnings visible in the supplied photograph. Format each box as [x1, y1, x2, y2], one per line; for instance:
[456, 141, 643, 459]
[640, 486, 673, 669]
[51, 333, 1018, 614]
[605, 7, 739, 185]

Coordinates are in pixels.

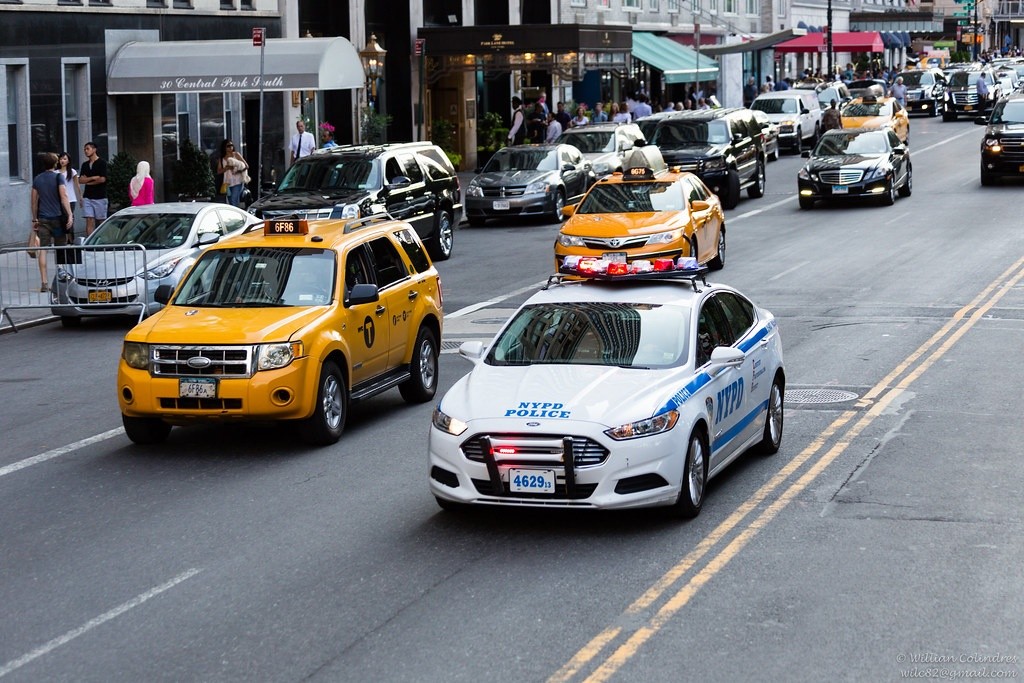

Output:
[107, 37, 366, 151]
[629, 31, 719, 84]
[775, 31, 911, 52]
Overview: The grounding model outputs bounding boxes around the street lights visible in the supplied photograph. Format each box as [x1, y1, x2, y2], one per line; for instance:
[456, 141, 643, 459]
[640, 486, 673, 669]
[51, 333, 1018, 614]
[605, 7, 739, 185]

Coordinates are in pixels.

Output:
[357, 31, 387, 114]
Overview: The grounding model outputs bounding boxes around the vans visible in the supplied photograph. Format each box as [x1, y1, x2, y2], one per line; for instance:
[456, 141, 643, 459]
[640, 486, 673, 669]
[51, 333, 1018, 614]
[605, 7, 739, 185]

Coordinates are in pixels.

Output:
[748, 89, 823, 155]
[794, 80, 853, 118]
[633, 106, 768, 211]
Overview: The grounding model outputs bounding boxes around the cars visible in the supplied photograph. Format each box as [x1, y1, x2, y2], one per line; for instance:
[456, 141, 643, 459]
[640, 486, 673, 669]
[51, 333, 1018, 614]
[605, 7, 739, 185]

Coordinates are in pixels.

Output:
[907, 55, 1024, 96]
[847, 78, 888, 101]
[550, 121, 649, 185]
[465, 143, 590, 226]
[840, 83, 910, 146]
[553, 145, 726, 281]
[51, 202, 265, 328]
[425, 254, 786, 520]
[797, 127, 913, 209]
[632, 111, 691, 145]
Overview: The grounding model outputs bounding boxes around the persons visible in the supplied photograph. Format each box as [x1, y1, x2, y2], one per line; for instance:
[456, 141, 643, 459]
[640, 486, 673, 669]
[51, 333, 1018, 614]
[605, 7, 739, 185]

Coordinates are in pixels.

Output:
[889, 76, 907, 108]
[744, 62, 902, 109]
[289, 121, 315, 164]
[54, 152, 84, 263]
[78, 141, 109, 238]
[217, 139, 249, 206]
[507, 96, 527, 146]
[528, 85, 720, 145]
[980, 45, 1024, 67]
[823, 99, 843, 130]
[319, 129, 338, 149]
[128, 160, 154, 206]
[31, 152, 74, 292]
[977, 73, 990, 116]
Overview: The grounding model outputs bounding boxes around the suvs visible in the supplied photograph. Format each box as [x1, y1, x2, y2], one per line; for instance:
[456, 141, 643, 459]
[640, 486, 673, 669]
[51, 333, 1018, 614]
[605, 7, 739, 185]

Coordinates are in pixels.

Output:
[248, 140, 463, 262]
[973, 94, 1024, 186]
[117, 211, 445, 446]
[941, 68, 1002, 122]
[888, 67, 948, 117]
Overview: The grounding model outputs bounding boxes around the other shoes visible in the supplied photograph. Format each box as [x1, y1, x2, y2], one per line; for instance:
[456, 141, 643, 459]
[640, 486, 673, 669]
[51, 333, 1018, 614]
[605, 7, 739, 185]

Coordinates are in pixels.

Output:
[41, 283, 51, 291]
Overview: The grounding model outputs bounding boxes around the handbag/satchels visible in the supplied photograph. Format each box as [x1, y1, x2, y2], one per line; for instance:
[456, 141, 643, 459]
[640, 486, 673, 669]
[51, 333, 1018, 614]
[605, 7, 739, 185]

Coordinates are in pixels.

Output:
[221, 183, 230, 194]
[27, 230, 40, 257]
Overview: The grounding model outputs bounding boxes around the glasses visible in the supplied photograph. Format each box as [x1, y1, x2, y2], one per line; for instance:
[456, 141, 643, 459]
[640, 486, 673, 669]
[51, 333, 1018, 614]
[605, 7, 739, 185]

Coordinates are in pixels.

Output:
[226, 144, 234, 147]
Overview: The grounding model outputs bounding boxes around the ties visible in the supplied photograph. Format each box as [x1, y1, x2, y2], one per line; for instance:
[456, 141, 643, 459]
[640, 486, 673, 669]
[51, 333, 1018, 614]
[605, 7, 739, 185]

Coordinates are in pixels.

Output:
[296, 135, 302, 158]
